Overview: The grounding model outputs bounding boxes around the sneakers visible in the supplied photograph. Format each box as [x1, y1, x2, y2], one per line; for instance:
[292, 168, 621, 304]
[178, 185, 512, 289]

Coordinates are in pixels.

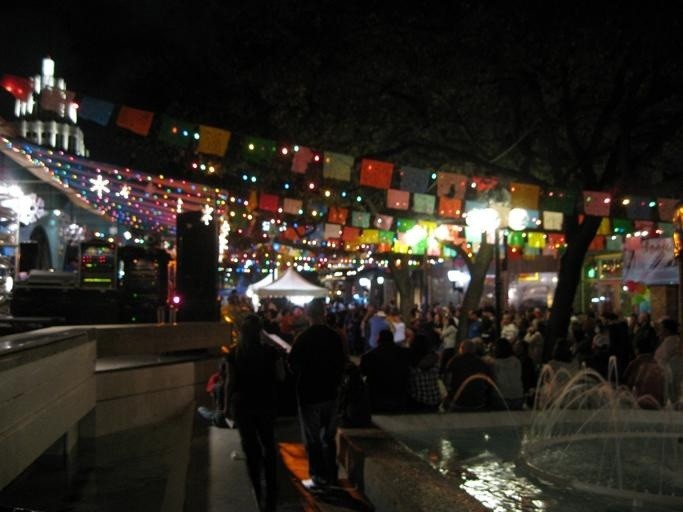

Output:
[302, 477, 341, 493]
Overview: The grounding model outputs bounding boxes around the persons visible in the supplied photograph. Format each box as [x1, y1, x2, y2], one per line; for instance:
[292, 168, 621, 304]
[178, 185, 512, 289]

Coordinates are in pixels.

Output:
[208, 289, 682, 492]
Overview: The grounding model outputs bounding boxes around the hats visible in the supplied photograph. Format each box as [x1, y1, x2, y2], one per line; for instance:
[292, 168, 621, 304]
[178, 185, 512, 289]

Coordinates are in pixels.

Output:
[306, 298, 324, 318]
[661, 319, 680, 334]
[594, 335, 610, 349]
